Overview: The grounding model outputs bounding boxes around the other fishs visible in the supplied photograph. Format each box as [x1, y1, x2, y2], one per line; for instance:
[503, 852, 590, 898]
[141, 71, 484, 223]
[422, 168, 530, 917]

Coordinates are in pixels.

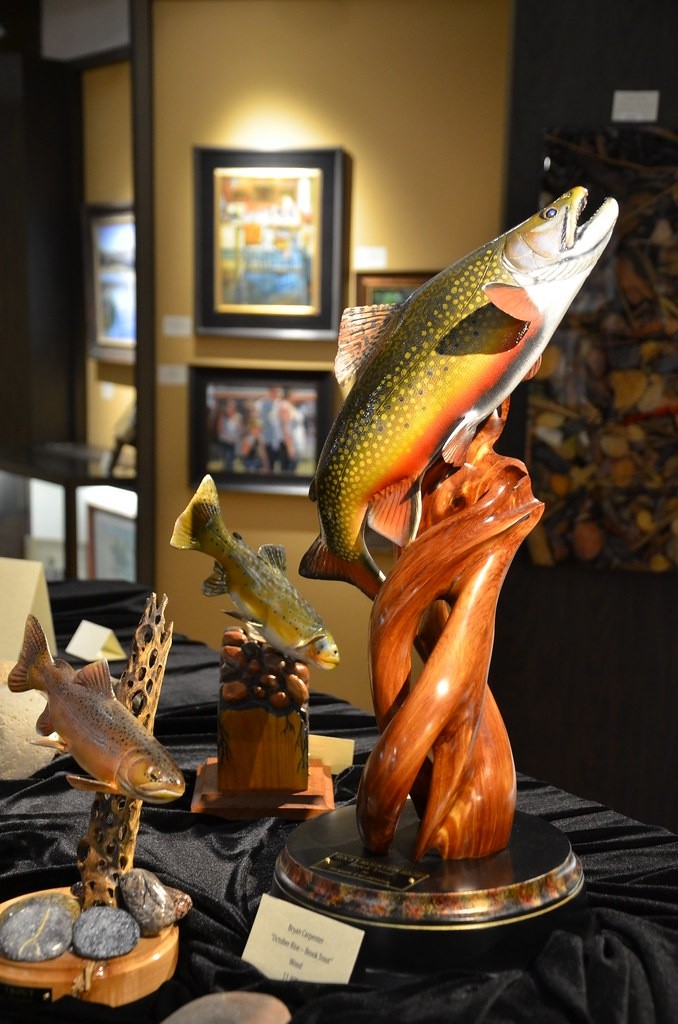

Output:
[168, 473, 340, 672]
[5, 614, 184, 805]
[298, 185, 621, 596]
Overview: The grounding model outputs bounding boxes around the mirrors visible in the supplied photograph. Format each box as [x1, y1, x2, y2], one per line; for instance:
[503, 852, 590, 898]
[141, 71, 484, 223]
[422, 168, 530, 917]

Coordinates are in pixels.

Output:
[0, 0, 160, 609]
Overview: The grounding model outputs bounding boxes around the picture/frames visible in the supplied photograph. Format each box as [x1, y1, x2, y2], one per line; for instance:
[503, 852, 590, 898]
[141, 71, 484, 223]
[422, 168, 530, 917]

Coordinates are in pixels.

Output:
[189, 141, 354, 340]
[185, 362, 333, 499]
[87, 504, 145, 585]
[76, 204, 142, 367]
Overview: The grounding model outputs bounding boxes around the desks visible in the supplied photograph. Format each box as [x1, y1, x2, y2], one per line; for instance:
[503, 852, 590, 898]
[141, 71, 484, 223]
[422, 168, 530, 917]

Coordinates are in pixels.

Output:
[0, 619, 678, 1024]
